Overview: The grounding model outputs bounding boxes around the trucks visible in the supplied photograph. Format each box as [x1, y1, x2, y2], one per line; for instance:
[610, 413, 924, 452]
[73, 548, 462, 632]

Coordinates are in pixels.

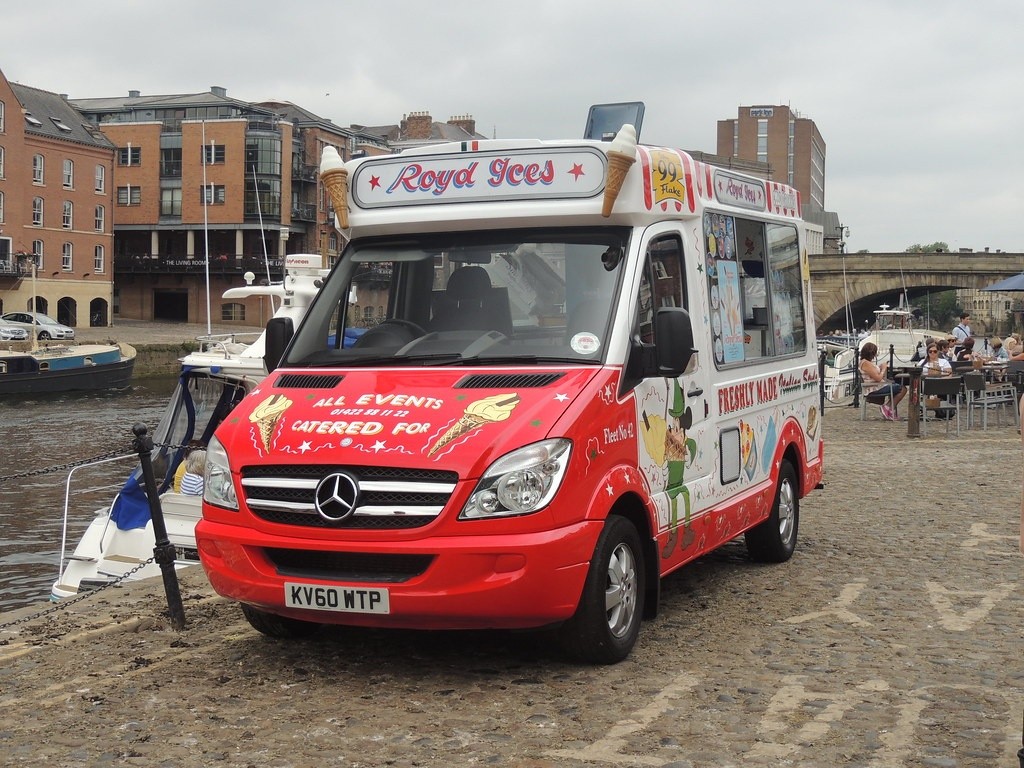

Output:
[194, 101, 824, 663]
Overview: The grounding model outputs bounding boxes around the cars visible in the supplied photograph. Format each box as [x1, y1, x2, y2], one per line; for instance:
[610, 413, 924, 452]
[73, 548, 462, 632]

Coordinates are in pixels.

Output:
[0, 312, 75, 340]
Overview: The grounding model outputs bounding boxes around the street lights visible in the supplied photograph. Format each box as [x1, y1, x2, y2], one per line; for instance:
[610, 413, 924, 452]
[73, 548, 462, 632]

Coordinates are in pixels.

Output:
[835, 223, 850, 254]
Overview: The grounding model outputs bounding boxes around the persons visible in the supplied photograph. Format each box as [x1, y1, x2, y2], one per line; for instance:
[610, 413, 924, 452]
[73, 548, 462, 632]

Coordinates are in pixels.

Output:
[885, 321, 896, 329]
[174, 440, 207, 493]
[914, 336, 1024, 421]
[858, 342, 907, 420]
[817, 327, 869, 338]
[180, 450, 206, 497]
[951, 313, 971, 356]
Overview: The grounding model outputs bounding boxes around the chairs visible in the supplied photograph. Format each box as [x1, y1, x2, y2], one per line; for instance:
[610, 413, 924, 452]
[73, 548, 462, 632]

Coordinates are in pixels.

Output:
[428, 263, 495, 332]
[857, 353, 1024, 437]
[564, 298, 640, 345]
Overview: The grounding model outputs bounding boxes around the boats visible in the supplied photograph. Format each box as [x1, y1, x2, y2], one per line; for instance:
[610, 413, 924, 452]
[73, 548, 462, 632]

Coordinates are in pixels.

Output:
[817, 257, 953, 402]
[0, 242, 137, 394]
[52, 120, 372, 603]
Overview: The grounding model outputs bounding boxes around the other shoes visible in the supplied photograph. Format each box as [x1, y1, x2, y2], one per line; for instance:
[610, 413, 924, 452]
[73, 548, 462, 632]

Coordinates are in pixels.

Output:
[881, 404, 898, 421]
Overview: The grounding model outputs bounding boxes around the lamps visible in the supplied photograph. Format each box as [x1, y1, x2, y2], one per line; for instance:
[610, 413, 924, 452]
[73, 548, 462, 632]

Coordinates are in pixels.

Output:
[84, 273, 90, 277]
[52, 271, 59, 276]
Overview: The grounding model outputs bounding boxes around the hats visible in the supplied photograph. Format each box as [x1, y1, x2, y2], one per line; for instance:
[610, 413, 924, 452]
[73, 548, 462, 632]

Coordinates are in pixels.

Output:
[947, 335, 958, 340]
[963, 337, 974, 345]
[990, 337, 1002, 347]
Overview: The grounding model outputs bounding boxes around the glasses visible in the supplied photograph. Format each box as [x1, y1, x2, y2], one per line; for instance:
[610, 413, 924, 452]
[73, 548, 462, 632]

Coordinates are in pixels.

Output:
[929, 350, 938, 354]
[950, 339, 957, 342]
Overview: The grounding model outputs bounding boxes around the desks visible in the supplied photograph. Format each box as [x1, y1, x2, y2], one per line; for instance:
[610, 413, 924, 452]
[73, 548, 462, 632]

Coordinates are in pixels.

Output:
[895, 372, 952, 422]
[983, 356, 996, 359]
[955, 364, 1008, 408]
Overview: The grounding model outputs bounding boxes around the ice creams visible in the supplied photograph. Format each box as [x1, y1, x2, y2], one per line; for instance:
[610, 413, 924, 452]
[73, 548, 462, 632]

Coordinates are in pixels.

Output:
[427, 392, 520, 458]
[601, 124, 637, 217]
[320, 145, 349, 230]
[248, 393, 293, 454]
[740, 418, 754, 466]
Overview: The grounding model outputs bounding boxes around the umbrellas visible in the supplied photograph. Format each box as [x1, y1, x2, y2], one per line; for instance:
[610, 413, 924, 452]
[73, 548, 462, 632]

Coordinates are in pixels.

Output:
[978, 273, 1024, 292]
